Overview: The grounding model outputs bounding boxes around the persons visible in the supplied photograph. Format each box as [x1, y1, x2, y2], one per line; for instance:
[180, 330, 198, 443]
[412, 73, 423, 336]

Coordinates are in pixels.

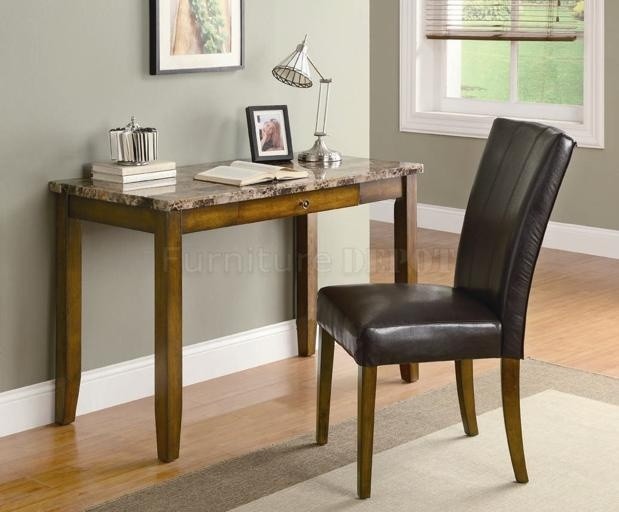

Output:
[260, 119, 282, 152]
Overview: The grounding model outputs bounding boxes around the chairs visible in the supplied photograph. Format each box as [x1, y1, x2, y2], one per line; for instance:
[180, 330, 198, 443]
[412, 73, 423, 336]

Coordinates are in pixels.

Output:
[315, 117, 576, 503]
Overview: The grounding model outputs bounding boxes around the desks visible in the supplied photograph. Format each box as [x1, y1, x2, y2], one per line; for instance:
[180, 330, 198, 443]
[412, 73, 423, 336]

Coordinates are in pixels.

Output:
[39, 147, 426, 463]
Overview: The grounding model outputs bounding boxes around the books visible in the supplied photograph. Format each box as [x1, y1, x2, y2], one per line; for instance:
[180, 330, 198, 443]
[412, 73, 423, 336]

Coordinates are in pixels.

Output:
[92, 161, 177, 192]
[192, 160, 309, 188]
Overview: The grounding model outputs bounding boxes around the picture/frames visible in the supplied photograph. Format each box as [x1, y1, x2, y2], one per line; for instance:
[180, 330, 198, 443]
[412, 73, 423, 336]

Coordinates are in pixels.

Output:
[146, 1, 247, 77]
[244, 102, 295, 163]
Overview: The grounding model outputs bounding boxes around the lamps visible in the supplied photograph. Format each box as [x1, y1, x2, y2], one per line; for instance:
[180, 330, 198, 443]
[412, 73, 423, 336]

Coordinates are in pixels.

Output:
[269, 33, 341, 167]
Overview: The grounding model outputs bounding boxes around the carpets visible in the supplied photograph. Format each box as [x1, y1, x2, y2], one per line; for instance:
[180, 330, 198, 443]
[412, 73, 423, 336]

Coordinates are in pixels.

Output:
[59, 348, 618, 512]
